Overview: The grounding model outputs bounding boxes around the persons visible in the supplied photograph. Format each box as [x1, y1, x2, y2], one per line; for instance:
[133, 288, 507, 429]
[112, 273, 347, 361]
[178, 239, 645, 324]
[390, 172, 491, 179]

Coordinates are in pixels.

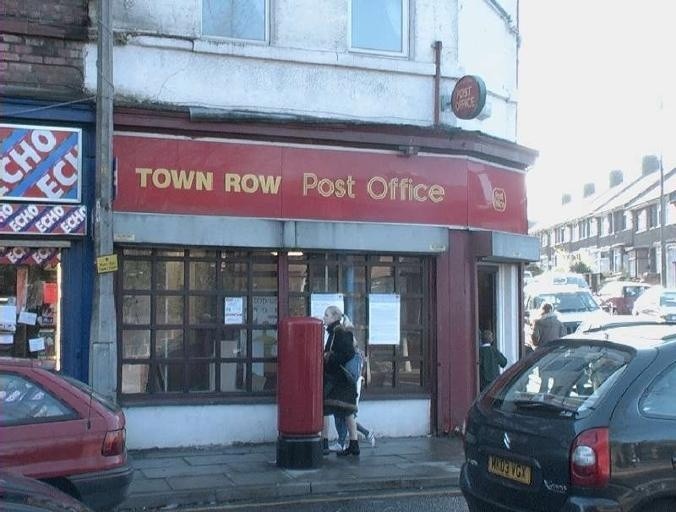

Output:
[191, 312, 213, 377]
[530, 302, 565, 394]
[640, 264, 652, 283]
[479, 329, 508, 393]
[322, 305, 377, 458]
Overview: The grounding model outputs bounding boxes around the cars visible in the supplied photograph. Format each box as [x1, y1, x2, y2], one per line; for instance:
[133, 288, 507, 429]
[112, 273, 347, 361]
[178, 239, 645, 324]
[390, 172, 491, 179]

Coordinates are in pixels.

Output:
[524, 262, 675, 355]
[459, 323, 675, 512]
[0, 356, 135, 512]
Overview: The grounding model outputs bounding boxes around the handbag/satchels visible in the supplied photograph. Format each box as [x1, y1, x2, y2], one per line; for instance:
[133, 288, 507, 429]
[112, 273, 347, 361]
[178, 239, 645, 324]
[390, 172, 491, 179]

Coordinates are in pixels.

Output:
[344, 349, 363, 384]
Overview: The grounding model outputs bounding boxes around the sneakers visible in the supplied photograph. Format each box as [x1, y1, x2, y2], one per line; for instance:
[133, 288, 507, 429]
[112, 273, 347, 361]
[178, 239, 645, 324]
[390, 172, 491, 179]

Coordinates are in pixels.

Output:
[367, 432, 375, 447]
[323, 443, 360, 455]
